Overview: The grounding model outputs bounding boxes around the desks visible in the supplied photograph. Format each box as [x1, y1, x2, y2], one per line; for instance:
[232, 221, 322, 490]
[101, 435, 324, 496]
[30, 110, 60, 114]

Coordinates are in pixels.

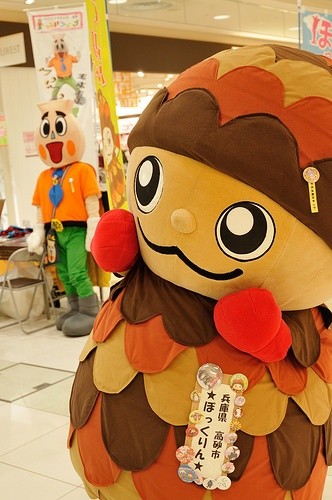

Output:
[0, 236, 67, 321]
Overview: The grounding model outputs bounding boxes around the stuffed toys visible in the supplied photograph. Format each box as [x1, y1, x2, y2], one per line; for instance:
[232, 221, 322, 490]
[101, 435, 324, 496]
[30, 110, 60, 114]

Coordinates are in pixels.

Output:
[40, 32, 88, 105]
[67, 45, 331, 499]
[27, 100, 102, 337]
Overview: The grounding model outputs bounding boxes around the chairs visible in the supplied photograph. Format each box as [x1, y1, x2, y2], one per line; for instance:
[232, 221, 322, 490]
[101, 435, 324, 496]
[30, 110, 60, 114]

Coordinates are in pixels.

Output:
[0, 248, 58, 334]
